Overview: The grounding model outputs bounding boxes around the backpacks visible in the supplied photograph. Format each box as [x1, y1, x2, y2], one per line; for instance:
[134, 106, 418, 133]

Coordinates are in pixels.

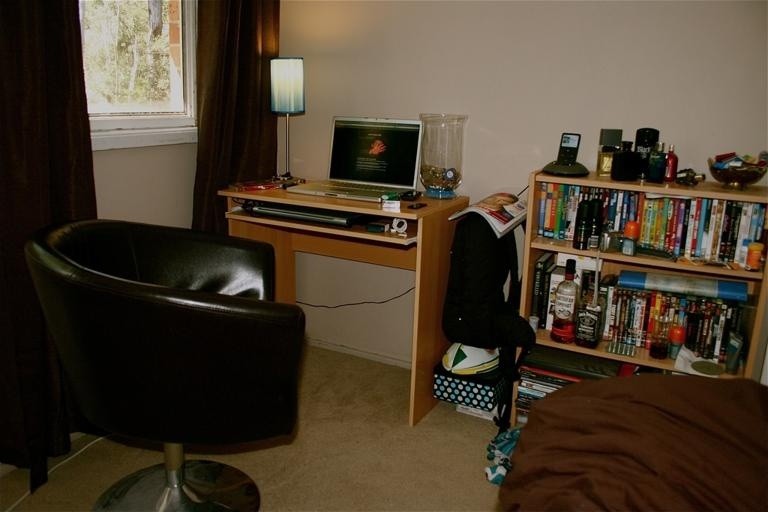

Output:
[443, 213, 536, 349]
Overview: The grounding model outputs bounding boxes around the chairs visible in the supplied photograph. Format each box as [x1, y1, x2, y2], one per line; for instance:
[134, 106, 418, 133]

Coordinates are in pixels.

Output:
[22, 219, 305, 512]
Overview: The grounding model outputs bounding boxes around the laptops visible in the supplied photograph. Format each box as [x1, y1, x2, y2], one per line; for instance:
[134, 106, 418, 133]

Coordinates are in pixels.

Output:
[286, 116, 425, 203]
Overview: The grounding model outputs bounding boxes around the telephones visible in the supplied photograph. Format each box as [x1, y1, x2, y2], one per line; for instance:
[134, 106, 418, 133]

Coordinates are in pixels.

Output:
[539, 132, 590, 178]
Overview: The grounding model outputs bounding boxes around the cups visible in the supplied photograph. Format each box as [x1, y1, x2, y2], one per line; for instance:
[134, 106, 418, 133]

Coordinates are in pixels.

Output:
[648, 315, 672, 361]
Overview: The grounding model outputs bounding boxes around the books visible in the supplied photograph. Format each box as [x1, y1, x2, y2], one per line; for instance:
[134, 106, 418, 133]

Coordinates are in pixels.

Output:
[539, 184, 765, 271]
[532, 251, 756, 375]
[448, 193, 528, 238]
[454, 402, 509, 423]
[517, 344, 667, 427]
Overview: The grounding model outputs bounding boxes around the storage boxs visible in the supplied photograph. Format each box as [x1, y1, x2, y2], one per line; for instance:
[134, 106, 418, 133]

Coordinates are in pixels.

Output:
[433, 360, 514, 412]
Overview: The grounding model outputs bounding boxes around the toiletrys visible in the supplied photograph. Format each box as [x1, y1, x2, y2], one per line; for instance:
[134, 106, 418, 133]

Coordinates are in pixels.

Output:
[646, 143, 666, 184]
[663, 144, 679, 183]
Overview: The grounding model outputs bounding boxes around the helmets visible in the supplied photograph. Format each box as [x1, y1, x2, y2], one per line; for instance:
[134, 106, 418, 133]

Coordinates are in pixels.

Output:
[441, 344, 500, 375]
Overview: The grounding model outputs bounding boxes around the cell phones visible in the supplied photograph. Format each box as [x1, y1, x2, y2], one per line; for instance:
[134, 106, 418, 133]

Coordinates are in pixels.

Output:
[402, 190, 422, 201]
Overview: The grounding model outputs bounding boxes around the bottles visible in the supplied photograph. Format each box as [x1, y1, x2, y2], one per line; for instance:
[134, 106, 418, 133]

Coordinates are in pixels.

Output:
[662, 143, 681, 184]
[550, 255, 583, 344]
[674, 167, 706, 187]
[609, 141, 644, 183]
[642, 140, 666, 184]
[743, 239, 765, 274]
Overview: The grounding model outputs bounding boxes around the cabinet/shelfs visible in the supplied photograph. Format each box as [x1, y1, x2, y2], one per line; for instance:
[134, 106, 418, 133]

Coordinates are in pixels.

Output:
[509, 168, 768, 427]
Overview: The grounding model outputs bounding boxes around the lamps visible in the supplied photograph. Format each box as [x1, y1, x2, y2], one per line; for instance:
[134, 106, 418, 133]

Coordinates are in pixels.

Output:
[270, 57, 305, 182]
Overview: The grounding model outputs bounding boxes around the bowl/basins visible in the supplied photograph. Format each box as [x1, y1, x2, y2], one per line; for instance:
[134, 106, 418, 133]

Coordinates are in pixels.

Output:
[708, 161, 768, 192]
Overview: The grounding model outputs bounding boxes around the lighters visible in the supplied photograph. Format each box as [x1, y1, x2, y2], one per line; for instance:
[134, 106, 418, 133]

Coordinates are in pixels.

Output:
[381, 191, 398, 200]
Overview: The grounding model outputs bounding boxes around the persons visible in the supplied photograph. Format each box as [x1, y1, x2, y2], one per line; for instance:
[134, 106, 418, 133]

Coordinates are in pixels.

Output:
[442, 216, 535, 432]
[361, 137, 387, 157]
[473, 192, 517, 224]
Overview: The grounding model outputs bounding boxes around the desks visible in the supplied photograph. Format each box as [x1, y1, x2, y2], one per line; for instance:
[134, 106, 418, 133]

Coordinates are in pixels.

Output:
[216, 177, 469, 426]
